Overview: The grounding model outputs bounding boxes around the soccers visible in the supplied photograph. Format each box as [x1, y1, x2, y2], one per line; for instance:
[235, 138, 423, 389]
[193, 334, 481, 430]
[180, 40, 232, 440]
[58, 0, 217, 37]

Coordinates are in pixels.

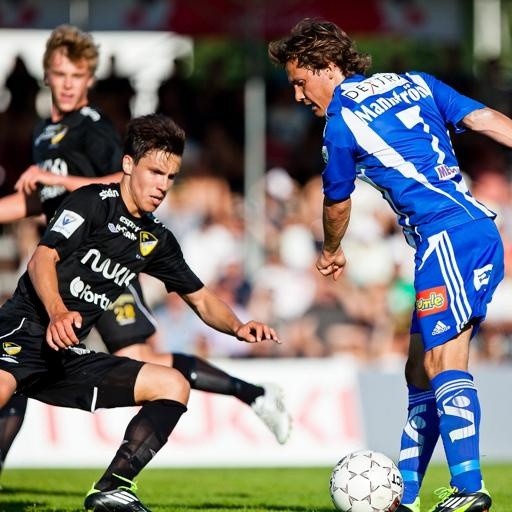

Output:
[329, 449, 404, 511]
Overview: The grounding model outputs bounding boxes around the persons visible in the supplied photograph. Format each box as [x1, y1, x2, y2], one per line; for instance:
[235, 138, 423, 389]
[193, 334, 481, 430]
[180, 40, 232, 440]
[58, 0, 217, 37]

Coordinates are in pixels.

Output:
[267, 19, 512, 512]
[474, 173, 512, 362]
[1, 217, 108, 355]
[0, 24, 292, 445]
[0, 115, 284, 512]
[140, 170, 415, 363]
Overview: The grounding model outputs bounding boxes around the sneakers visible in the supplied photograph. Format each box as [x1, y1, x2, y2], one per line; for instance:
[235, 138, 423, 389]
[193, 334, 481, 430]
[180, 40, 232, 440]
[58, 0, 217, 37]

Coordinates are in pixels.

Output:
[249, 382, 291, 444]
[395, 479, 491, 512]
[83, 481, 152, 512]
